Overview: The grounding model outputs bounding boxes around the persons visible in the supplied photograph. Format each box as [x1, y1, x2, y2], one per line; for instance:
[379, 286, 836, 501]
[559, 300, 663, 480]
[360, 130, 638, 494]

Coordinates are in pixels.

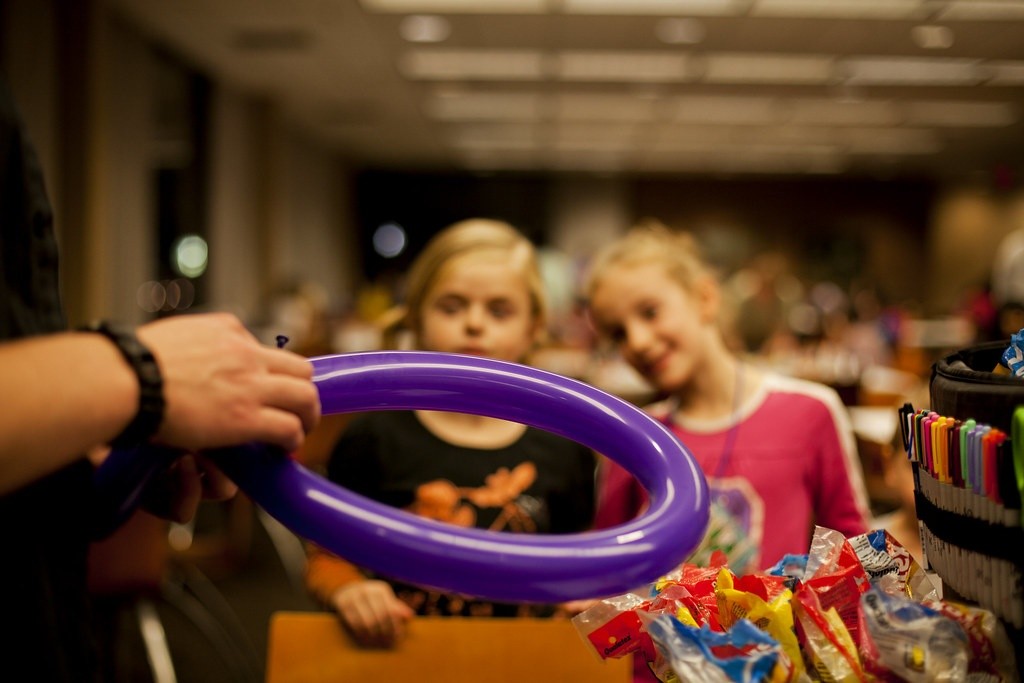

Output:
[577, 218, 870, 605]
[0, 78, 320, 683]
[761, 268, 1023, 411]
[305, 215, 598, 646]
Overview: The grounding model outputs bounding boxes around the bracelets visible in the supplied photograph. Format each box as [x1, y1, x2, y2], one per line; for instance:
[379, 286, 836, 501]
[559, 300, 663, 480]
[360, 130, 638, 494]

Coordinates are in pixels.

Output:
[73, 315, 166, 474]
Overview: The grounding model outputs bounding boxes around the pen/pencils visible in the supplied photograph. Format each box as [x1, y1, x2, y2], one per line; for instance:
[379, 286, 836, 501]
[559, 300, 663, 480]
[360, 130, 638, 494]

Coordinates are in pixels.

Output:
[907, 408, 1023, 628]
[898, 403, 915, 452]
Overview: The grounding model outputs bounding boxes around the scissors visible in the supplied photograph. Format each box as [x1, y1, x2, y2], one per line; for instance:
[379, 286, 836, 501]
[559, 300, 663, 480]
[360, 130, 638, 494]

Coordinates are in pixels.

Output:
[1011, 409, 1024, 492]
